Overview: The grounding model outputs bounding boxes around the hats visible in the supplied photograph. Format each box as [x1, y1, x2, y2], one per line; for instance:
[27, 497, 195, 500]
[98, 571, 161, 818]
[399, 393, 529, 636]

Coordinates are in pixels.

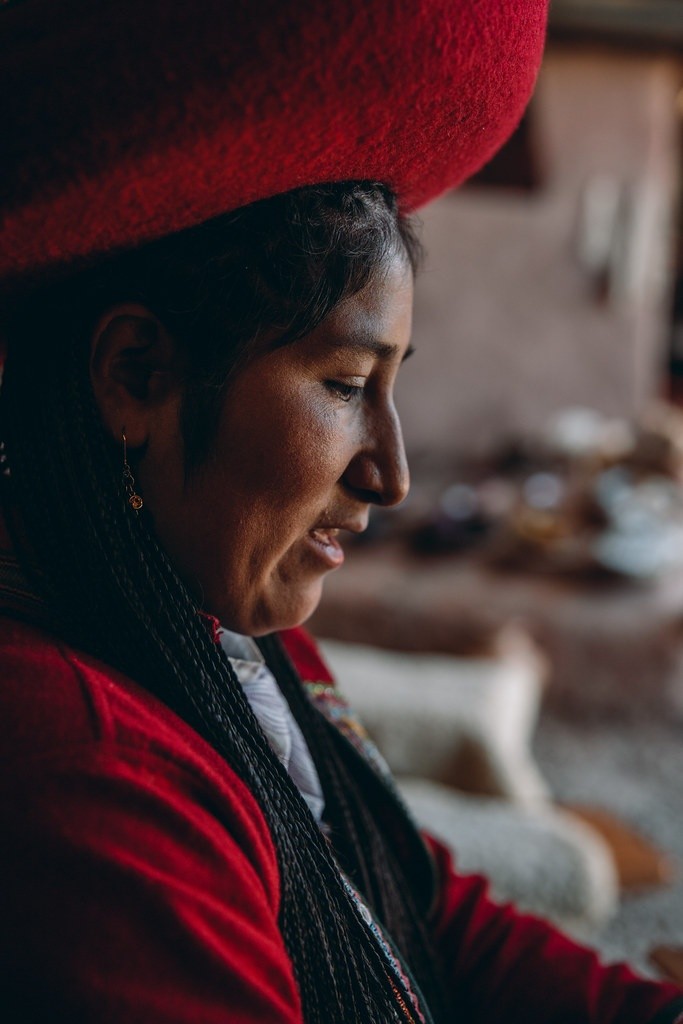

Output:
[0, 0, 550, 264]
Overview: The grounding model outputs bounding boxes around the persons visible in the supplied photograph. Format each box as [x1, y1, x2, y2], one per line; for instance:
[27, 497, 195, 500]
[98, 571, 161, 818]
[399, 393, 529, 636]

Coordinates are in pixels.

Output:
[0, 0, 683, 1024]
